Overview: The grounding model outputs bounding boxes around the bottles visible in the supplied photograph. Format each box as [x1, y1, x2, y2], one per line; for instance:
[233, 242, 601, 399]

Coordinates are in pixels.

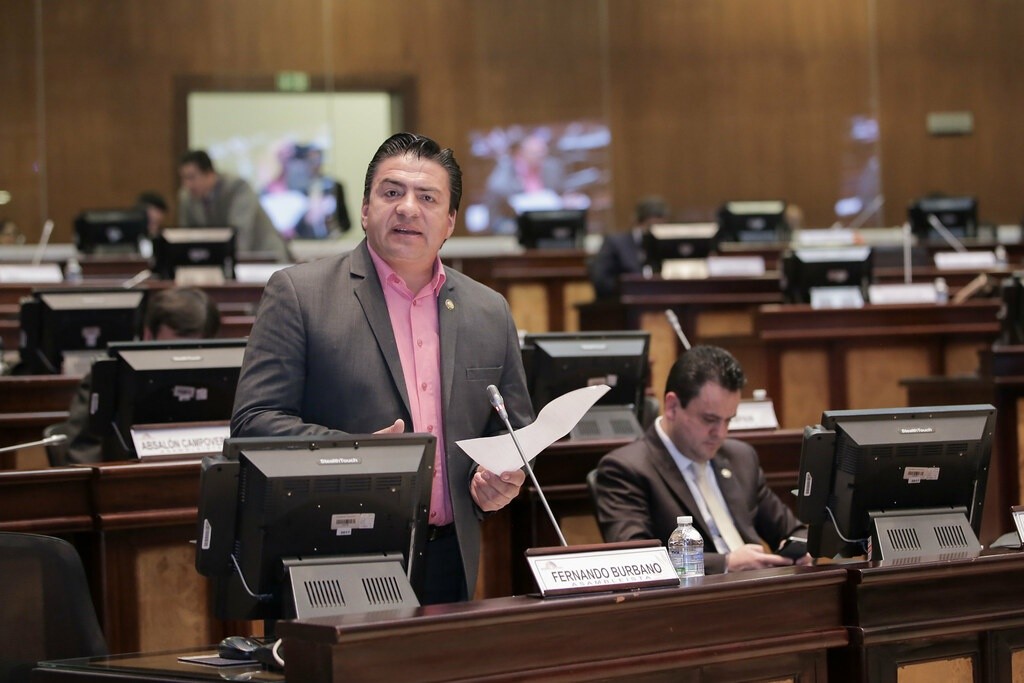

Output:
[65, 258, 81, 282]
[935, 278, 949, 304]
[667, 516, 705, 578]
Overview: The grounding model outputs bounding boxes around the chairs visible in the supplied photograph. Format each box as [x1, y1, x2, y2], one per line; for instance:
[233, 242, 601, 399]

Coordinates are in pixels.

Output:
[1, 533, 104, 682]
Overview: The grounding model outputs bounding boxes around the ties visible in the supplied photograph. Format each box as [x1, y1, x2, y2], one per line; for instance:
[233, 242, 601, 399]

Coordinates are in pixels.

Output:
[690, 460, 744, 552]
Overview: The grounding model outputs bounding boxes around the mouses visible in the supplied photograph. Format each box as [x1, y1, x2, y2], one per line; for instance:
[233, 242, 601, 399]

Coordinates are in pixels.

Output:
[218, 635, 264, 659]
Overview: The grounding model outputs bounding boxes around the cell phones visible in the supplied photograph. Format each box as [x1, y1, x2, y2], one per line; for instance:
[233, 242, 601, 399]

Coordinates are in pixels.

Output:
[774, 536, 808, 568]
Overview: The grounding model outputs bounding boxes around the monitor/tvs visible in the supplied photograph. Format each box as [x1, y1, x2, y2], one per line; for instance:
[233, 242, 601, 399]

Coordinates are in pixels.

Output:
[20, 195, 1023, 624]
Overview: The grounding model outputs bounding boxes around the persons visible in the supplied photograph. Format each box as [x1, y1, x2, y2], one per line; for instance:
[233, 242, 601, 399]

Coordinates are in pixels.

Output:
[598, 345, 812, 579]
[134, 192, 174, 278]
[175, 152, 287, 263]
[589, 205, 664, 322]
[232, 131, 538, 600]
[297, 145, 350, 239]
[63, 287, 219, 463]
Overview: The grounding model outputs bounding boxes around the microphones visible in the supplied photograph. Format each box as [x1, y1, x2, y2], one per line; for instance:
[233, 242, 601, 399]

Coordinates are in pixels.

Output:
[852, 197, 883, 229]
[664, 308, 691, 350]
[32, 220, 53, 265]
[0, 434, 67, 454]
[486, 384, 567, 547]
[928, 214, 967, 252]
[901, 222, 913, 283]
[125, 269, 151, 288]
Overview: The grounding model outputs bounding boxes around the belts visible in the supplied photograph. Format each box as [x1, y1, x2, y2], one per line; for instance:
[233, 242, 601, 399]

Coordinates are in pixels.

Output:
[425, 523, 455, 543]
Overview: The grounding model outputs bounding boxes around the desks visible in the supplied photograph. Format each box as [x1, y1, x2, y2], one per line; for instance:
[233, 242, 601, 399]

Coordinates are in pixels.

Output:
[0, 231, 1024, 683]
